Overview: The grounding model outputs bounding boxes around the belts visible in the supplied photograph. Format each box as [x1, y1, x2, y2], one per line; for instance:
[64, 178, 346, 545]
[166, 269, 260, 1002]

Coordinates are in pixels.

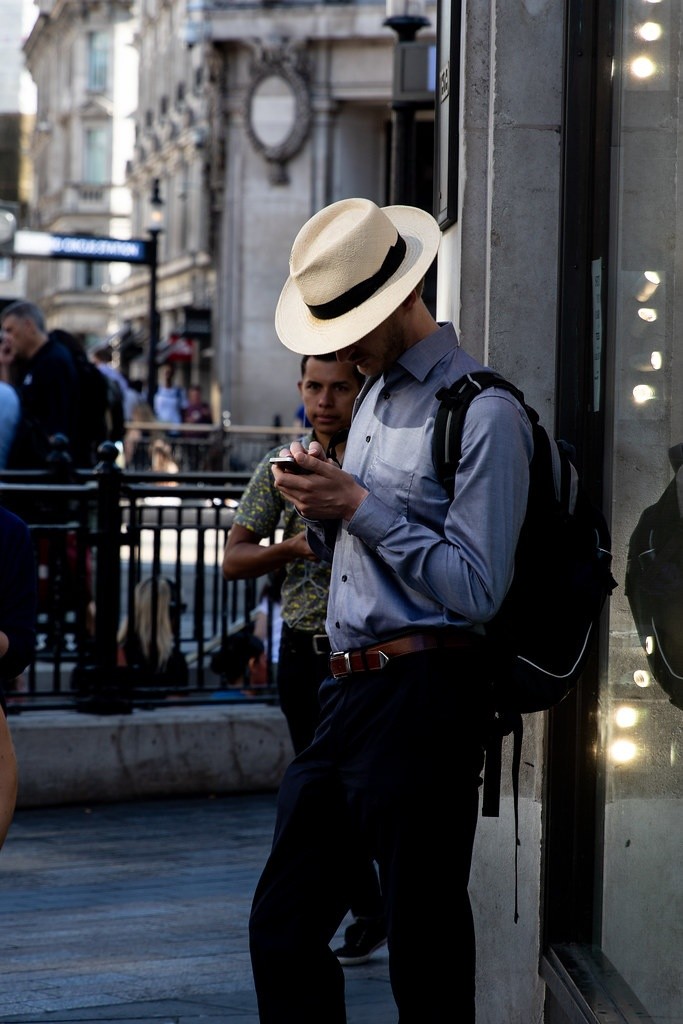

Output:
[327, 634, 434, 680]
[279, 626, 329, 653]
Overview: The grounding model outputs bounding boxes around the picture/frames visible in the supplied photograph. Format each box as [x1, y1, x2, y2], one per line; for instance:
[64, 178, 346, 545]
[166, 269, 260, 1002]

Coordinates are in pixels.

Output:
[434, 0, 461, 230]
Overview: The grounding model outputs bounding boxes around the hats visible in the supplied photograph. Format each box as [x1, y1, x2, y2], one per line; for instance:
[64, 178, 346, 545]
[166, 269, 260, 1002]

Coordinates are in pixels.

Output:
[274, 197, 441, 357]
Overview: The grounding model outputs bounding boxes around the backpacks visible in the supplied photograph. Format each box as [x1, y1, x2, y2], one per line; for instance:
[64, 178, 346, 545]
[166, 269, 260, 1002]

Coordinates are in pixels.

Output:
[430, 368, 609, 711]
[622, 447, 681, 708]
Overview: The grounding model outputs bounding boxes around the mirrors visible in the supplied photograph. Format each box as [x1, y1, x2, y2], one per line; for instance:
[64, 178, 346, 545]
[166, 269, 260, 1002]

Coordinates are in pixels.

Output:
[241, 34, 311, 187]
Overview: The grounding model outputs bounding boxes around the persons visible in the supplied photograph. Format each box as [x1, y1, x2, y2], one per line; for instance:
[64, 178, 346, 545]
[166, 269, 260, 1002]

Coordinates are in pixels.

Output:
[249, 199, 539, 1024]
[209, 633, 271, 699]
[0, 300, 129, 849]
[114, 571, 193, 700]
[223, 351, 388, 965]
[90, 342, 214, 472]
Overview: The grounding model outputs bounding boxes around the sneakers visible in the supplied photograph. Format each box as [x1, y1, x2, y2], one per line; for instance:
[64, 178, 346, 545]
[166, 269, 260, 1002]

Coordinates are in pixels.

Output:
[333, 915, 393, 963]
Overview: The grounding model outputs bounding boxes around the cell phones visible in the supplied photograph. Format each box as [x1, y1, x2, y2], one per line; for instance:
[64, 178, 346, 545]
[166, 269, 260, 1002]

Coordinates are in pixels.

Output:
[269, 457, 306, 476]
